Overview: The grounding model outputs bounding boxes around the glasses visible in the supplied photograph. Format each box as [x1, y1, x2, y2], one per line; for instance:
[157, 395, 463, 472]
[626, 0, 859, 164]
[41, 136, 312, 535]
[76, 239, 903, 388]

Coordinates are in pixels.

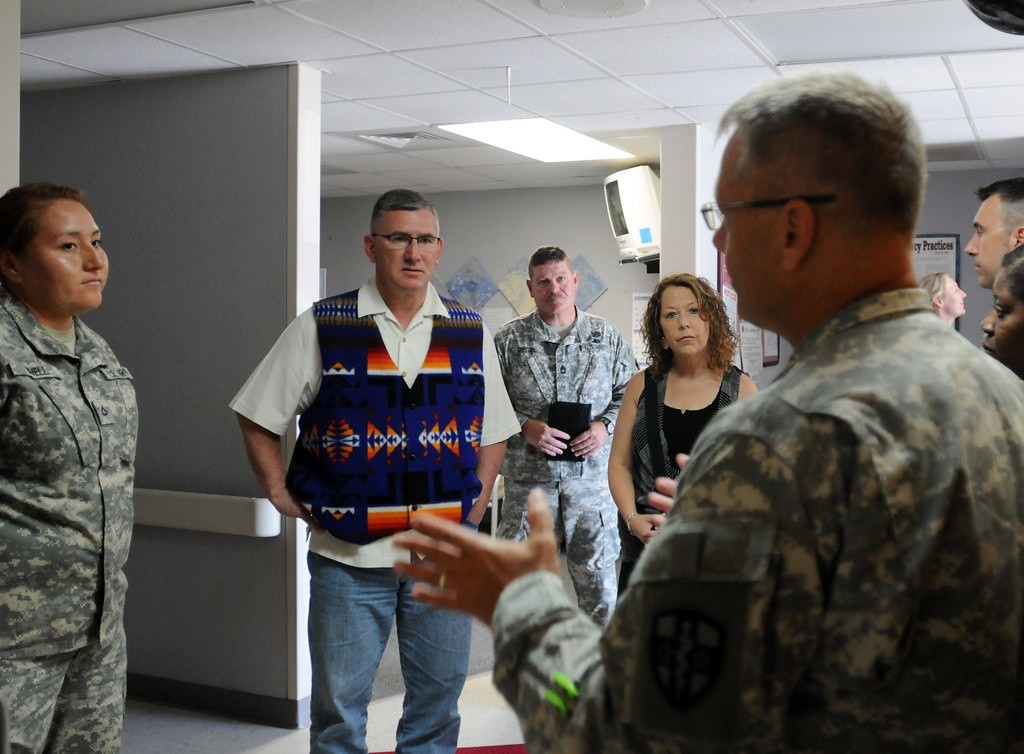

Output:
[372, 231, 441, 251]
[701, 189, 838, 230]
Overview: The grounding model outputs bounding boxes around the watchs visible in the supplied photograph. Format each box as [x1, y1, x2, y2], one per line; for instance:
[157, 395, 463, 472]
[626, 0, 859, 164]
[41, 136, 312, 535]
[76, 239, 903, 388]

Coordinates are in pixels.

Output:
[599, 416, 615, 435]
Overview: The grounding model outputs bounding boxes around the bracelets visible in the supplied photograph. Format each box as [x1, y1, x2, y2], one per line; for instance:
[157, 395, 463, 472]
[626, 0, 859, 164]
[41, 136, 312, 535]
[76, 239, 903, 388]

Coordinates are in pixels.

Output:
[624, 512, 637, 534]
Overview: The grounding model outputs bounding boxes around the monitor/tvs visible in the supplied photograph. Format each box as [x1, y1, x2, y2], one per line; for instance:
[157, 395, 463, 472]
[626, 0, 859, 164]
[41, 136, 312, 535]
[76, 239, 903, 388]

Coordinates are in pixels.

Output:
[604, 165, 662, 255]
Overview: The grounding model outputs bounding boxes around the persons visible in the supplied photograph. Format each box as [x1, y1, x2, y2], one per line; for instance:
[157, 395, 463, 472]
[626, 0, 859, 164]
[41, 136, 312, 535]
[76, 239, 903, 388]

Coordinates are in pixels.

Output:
[227, 189, 522, 754]
[392, 71, 1024, 754]
[0, 182, 138, 753]
[607, 272, 758, 599]
[495, 246, 639, 631]
